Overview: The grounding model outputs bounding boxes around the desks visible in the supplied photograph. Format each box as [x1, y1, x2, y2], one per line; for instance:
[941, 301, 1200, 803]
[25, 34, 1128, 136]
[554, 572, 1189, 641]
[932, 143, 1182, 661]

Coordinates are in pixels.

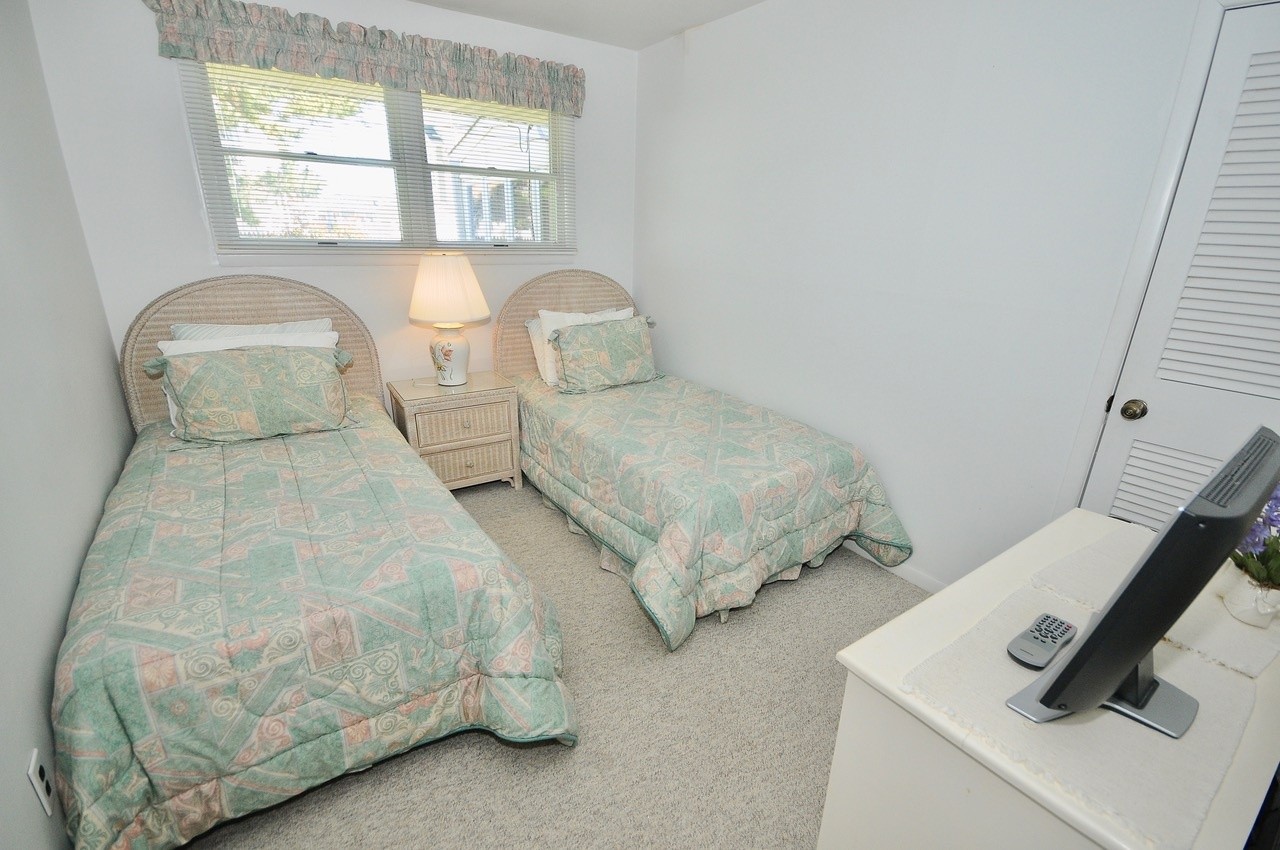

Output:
[818, 507, 1280, 849]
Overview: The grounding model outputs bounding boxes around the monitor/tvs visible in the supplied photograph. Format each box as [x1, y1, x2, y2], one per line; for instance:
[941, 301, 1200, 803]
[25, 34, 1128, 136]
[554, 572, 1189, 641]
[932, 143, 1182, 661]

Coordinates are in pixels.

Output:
[1006, 424, 1279, 740]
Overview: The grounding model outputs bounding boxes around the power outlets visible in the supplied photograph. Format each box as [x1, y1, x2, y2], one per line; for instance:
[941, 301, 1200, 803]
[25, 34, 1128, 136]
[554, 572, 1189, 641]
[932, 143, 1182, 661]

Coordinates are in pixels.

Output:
[28, 748, 56, 816]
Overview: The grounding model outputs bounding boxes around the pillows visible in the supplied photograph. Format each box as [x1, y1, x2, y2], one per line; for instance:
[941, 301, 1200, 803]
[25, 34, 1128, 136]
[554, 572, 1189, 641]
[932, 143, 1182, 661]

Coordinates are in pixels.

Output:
[143, 345, 366, 451]
[525, 308, 616, 386]
[171, 316, 332, 341]
[541, 307, 635, 384]
[548, 314, 664, 393]
[158, 331, 339, 437]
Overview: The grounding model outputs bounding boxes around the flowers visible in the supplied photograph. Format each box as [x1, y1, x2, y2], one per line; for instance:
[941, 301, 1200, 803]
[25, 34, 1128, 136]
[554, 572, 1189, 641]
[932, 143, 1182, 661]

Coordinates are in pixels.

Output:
[1231, 482, 1280, 591]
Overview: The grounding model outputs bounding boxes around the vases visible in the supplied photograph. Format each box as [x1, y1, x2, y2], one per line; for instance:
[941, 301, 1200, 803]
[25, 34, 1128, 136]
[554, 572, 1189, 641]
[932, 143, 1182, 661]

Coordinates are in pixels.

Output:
[1215, 561, 1280, 631]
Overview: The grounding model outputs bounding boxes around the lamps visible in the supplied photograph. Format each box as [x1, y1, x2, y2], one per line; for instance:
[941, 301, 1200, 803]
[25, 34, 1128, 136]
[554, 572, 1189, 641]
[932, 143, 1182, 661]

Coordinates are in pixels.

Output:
[410, 251, 490, 386]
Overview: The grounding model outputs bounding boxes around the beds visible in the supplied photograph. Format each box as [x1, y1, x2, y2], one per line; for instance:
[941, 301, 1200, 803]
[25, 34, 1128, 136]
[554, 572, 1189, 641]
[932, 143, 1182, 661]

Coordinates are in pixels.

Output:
[53, 274, 574, 850]
[494, 268, 911, 654]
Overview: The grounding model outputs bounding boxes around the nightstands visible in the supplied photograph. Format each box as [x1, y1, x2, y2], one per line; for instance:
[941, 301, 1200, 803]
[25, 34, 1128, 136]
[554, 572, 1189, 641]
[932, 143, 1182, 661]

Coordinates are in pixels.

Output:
[385, 371, 522, 490]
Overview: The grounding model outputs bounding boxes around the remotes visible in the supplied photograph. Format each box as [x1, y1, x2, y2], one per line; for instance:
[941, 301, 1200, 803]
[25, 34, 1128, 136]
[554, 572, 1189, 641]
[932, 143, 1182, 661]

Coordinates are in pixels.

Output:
[1006, 613, 1078, 669]
